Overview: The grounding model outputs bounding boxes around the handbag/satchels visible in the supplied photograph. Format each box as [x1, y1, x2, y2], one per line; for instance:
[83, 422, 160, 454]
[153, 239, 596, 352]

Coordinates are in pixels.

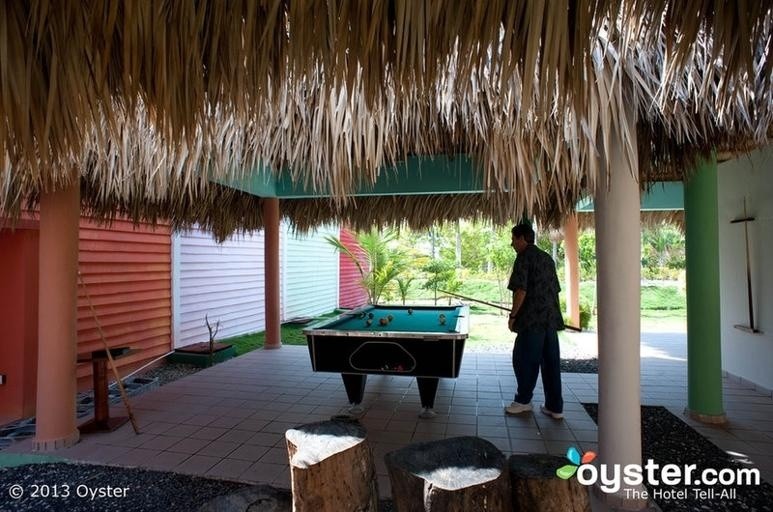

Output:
[509, 313, 516, 319]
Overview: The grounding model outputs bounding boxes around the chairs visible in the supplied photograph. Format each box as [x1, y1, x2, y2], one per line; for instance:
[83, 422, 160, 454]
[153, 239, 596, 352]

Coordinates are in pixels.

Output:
[539, 402, 564, 419]
[503, 401, 535, 414]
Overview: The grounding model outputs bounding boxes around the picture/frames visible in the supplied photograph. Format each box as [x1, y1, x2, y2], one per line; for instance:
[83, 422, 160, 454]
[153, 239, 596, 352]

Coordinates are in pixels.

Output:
[74, 347, 131, 436]
[302, 304, 471, 417]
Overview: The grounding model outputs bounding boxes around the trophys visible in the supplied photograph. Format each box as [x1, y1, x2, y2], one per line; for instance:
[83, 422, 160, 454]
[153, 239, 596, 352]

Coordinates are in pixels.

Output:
[743, 197, 754, 329]
[77, 272, 143, 435]
[437, 289, 582, 331]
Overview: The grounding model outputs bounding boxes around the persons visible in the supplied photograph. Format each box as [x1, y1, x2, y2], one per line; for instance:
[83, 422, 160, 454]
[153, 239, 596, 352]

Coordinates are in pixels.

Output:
[505, 223, 565, 418]
